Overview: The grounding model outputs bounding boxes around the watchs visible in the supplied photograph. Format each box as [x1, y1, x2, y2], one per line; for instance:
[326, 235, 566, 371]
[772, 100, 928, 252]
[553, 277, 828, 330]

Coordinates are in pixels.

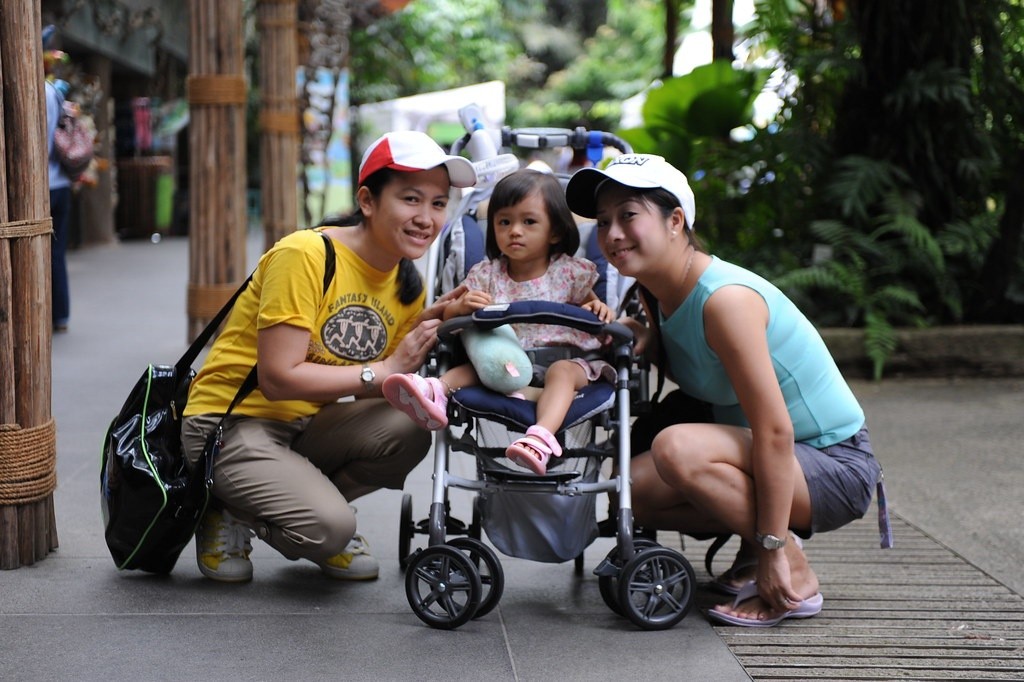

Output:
[360, 363, 376, 392]
[755, 529, 787, 551]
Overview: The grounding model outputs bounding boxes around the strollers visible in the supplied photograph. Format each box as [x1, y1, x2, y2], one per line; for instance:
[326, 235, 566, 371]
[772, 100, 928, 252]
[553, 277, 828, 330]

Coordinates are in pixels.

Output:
[398, 123, 697, 630]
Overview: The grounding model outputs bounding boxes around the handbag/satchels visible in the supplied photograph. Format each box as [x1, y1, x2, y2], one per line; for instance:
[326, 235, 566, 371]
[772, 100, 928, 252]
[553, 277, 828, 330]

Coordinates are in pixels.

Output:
[629, 388, 712, 452]
[97, 366, 213, 580]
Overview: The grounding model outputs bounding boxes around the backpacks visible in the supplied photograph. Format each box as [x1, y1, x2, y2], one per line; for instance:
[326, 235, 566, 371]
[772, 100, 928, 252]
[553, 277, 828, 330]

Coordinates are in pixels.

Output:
[47, 78, 98, 179]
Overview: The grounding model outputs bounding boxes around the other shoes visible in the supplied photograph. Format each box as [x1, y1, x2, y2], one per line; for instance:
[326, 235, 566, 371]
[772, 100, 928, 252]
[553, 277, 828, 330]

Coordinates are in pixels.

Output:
[51, 323, 70, 336]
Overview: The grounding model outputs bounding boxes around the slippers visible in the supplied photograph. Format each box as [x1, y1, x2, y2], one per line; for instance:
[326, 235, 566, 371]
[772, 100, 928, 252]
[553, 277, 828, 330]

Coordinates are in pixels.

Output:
[711, 529, 805, 594]
[708, 581, 826, 627]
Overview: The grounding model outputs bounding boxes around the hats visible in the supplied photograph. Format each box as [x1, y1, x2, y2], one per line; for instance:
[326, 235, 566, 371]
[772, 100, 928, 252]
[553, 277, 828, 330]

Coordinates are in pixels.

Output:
[360, 129, 478, 189]
[566, 154, 697, 230]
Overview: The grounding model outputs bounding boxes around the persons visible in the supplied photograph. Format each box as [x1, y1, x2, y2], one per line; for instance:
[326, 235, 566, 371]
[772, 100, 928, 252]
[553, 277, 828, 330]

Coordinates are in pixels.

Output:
[181, 129, 477, 582]
[382, 169, 612, 476]
[44, 78, 73, 331]
[565, 154, 894, 628]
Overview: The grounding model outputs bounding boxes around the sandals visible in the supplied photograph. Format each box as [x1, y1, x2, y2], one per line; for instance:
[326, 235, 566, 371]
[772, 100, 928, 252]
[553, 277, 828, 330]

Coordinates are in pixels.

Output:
[381, 373, 449, 430]
[505, 424, 563, 476]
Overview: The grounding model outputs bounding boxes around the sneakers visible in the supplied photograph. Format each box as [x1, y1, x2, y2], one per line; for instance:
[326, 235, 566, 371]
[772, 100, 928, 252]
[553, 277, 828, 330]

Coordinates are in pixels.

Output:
[314, 531, 381, 580]
[195, 503, 257, 583]
